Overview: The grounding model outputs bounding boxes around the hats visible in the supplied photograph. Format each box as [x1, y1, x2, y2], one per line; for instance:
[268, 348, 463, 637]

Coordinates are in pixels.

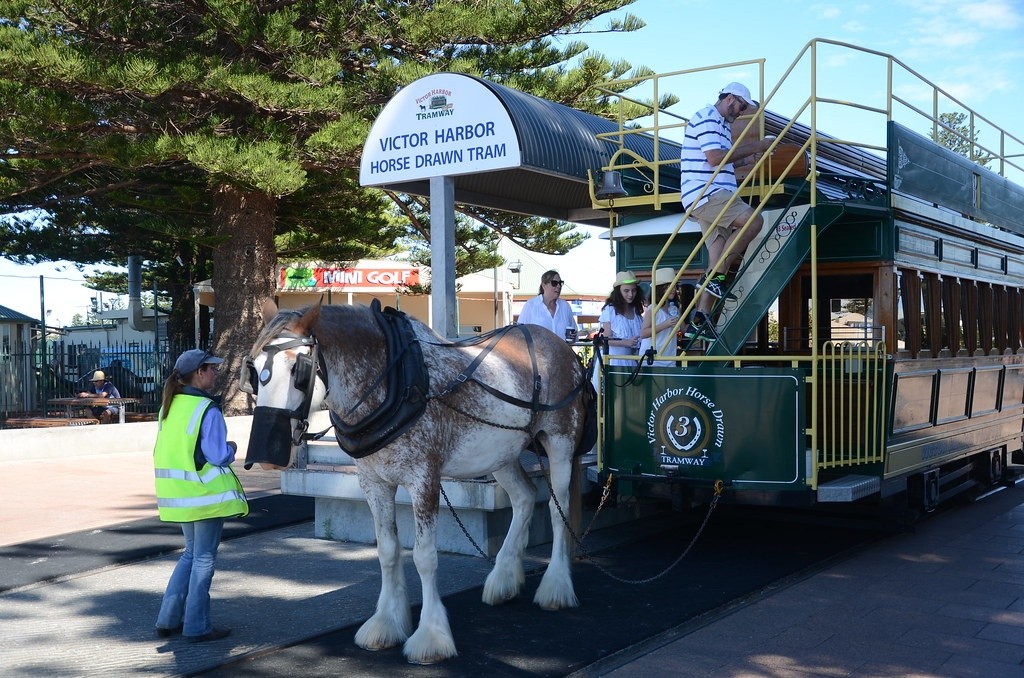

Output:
[613, 271, 640, 287]
[650, 268, 682, 287]
[89, 371, 108, 382]
[173, 349, 224, 378]
[719, 82, 757, 108]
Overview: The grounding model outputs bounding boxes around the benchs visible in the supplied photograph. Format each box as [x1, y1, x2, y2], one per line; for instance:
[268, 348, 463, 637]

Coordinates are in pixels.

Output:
[99, 411, 158, 424]
[730, 100, 887, 198]
[5, 415, 100, 428]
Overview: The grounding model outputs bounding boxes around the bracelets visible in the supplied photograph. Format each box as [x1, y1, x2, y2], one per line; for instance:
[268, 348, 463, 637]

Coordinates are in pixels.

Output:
[86, 394, 89, 397]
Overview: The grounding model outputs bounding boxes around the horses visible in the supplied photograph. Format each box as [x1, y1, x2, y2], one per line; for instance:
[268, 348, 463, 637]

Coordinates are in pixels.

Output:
[249, 294, 590, 665]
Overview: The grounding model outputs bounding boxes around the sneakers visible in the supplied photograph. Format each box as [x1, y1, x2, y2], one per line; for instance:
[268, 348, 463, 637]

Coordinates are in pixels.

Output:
[695, 273, 738, 301]
[684, 321, 719, 341]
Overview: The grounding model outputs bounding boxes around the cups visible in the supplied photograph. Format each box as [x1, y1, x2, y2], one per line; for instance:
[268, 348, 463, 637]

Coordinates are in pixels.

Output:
[765, 136, 776, 155]
[565, 326, 576, 342]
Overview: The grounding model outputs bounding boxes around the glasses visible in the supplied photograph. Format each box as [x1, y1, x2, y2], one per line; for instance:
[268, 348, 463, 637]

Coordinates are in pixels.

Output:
[551, 280, 564, 287]
[735, 97, 747, 110]
[197, 350, 213, 374]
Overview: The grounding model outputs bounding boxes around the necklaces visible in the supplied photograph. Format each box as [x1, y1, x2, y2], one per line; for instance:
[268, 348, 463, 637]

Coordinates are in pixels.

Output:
[623, 311, 634, 316]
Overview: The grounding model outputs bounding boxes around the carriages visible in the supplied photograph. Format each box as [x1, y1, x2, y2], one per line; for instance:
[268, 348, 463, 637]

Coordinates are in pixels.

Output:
[239, 38, 1024, 664]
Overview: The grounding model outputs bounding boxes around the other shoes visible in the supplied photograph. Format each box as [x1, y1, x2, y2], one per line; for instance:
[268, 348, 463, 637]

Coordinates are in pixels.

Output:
[156, 621, 184, 638]
[188, 627, 231, 643]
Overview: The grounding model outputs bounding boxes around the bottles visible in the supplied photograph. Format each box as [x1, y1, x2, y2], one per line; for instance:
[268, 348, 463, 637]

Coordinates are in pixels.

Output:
[668, 302, 680, 331]
[632, 316, 642, 348]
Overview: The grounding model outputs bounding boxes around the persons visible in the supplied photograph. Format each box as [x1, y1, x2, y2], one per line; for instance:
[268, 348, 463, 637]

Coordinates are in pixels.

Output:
[517, 270, 579, 349]
[79, 371, 121, 425]
[680, 82, 781, 342]
[153, 349, 250, 643]
[676, 283, 697, 349]
[591, 270, 648, 395]
[638, 267, 686, 367]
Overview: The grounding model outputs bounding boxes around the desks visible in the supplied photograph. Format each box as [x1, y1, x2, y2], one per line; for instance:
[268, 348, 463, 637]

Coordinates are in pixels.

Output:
[47, 397, 142, 424]
